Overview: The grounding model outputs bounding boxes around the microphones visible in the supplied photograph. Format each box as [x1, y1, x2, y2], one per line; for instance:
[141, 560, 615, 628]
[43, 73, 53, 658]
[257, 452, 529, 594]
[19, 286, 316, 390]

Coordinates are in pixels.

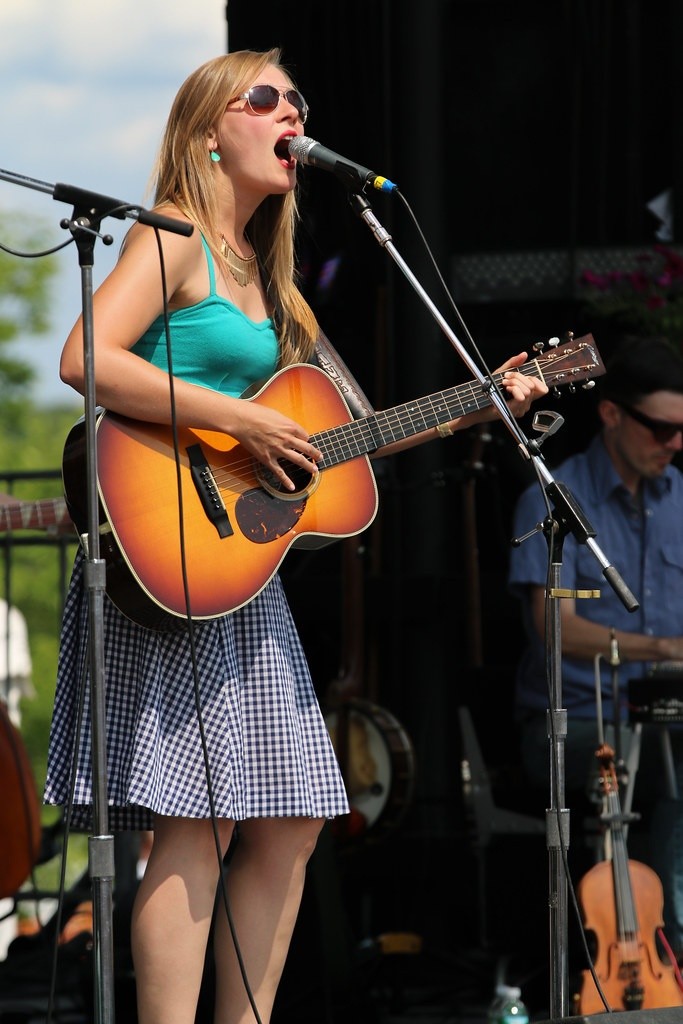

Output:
[287, 135, 401, 195]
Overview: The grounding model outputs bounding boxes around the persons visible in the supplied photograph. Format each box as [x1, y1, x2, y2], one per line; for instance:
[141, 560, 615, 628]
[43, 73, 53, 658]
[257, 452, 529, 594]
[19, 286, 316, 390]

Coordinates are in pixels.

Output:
[43, 48, 549, 1024]
[502, 341, 683, 1024]
[1, 595, 38, 938]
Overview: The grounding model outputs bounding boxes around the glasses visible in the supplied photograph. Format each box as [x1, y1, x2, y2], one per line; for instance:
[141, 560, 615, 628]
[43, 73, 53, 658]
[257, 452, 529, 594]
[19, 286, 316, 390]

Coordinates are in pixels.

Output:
[612, 399, 683, 445]
[224, 84, 309, 125]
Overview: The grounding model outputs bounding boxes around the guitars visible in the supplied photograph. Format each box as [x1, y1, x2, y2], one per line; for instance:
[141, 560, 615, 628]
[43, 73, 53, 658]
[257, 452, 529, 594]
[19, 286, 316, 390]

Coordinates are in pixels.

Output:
[567, 744, 682, 1017]
[60, 329, 608, 635]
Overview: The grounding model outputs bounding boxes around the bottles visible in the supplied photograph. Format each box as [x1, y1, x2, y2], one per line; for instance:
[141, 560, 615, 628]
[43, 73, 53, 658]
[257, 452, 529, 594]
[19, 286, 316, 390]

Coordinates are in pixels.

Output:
[488, 985, 510, 1024]
[500, 987, 529, 1024]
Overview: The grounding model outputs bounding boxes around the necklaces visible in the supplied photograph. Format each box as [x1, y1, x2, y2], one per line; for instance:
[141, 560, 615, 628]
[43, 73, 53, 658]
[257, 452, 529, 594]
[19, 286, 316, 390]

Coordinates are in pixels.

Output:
[220, 230, 258, 288]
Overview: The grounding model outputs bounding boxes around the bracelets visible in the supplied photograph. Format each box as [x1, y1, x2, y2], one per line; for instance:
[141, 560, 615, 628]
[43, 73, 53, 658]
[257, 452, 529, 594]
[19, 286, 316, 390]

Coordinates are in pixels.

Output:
[436, 422, 453, 438]
